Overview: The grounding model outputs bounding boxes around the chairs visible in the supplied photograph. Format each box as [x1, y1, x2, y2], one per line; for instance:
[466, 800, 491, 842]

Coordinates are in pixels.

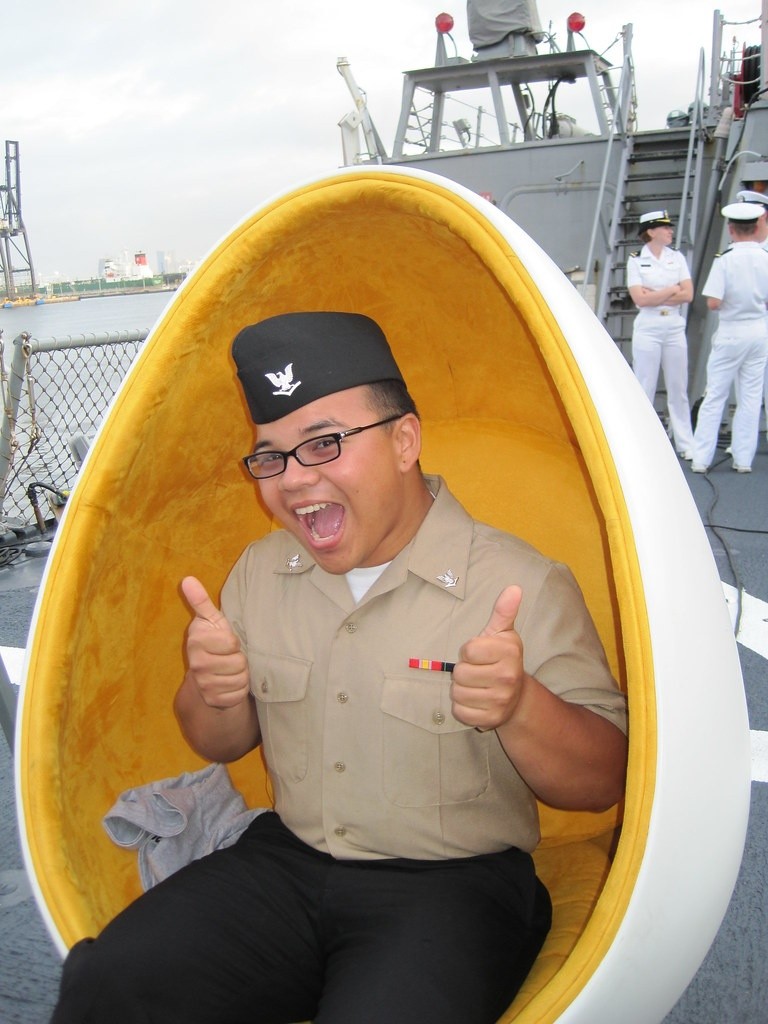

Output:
[14, 163, 752, 1024]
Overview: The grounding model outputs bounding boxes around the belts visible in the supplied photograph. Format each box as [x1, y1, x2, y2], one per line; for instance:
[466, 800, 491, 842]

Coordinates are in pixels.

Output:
[639, 309, 679, 315]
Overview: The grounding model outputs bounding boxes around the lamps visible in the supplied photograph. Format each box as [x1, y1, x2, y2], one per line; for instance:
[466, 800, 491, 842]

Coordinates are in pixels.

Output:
[454, 119, 472, 149]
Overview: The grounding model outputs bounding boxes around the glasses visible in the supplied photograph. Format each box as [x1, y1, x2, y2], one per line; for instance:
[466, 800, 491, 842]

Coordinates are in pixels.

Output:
[243, 414, 402, 479]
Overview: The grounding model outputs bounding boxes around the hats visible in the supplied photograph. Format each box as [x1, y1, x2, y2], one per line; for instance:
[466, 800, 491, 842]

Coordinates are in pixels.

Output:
[720, 203, 766, 225]
[737, 191, 768, 207]
[638, 209, 676, 235]
[232, 311, 407, 425]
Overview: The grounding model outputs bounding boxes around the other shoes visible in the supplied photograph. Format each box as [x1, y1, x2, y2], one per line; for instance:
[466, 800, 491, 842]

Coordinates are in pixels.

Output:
[690, 462, 707, 473]
[732, 461, 752, 473]
[681, 450, 693, 461]
[726, 446, 732, 453]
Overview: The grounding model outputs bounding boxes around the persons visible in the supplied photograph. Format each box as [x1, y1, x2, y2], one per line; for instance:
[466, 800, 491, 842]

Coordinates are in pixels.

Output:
[691, 189, 768, 475]
[624, 208, 695, 461]
[47, 310, 631, 1024]
